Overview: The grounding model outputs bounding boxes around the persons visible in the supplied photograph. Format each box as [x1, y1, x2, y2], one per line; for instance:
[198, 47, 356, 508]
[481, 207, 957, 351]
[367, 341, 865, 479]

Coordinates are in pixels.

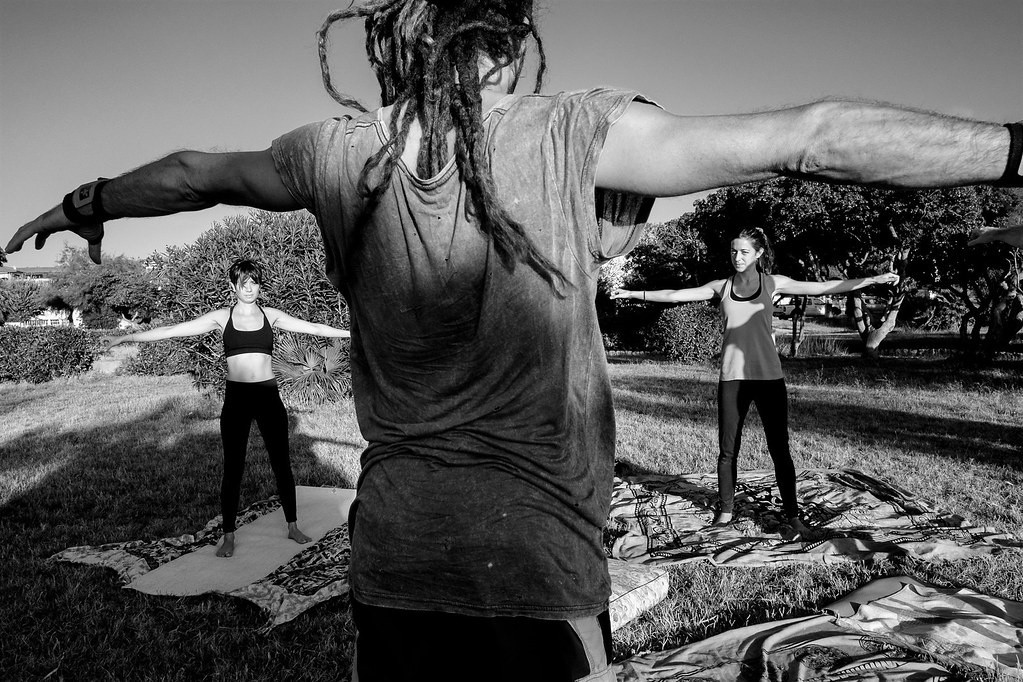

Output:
[99, 260, 350, 558]
[5, 0, 1023, 682]
[610, 227, 900, 532]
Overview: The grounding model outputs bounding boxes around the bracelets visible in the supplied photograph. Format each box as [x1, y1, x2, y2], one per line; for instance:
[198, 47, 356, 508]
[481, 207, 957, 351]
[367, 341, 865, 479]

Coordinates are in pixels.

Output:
[644, 290, 646, 301]
[132, 333, 135, 342]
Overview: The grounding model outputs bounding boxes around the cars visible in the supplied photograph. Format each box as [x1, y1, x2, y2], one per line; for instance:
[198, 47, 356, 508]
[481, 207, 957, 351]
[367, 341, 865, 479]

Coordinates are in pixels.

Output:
[861, 296, 887, 311]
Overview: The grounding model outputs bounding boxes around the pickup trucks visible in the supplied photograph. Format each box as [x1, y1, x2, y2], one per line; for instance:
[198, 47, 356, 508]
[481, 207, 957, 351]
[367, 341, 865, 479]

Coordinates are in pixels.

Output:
[775, 296, 828, 316]
